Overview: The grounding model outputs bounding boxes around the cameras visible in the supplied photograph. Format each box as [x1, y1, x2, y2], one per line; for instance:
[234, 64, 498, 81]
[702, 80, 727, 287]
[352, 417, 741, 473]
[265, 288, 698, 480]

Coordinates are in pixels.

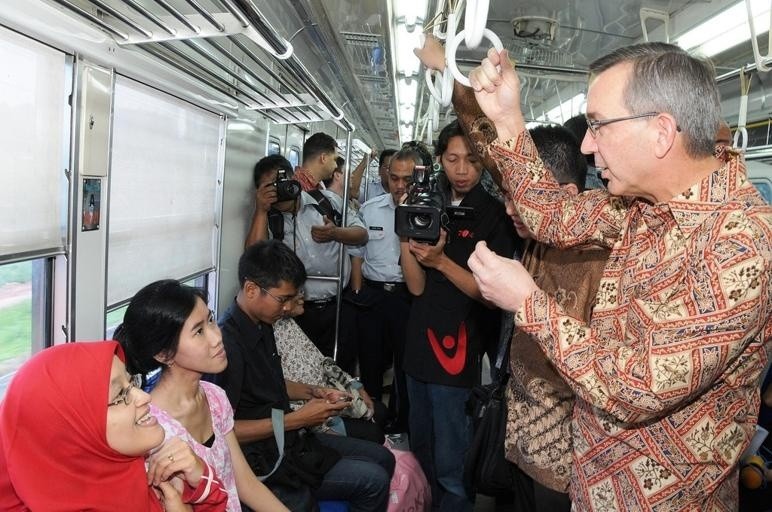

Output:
[265, 169, 302, 204]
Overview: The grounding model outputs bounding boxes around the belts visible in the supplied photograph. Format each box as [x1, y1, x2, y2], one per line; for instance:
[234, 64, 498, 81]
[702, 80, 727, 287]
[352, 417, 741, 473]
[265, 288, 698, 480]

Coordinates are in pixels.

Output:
[305, 295, 337, 309]
[363, 277, 407, 291]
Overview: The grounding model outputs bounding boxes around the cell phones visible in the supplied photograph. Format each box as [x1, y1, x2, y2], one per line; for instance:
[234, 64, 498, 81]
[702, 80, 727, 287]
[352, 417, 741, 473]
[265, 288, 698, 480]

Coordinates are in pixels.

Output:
[327, 397, 351, 404]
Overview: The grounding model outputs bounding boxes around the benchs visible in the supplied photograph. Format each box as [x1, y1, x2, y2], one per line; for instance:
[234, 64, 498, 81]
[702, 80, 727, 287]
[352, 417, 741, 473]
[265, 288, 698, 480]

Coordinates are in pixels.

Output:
[145, 370, 349, 512]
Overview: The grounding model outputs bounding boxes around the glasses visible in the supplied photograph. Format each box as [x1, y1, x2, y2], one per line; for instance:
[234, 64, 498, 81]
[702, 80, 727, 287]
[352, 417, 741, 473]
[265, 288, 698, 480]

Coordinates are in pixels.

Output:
[585, 111, 682, 135]
[249, 279, 295, 304]
[107, 372, 143, 407]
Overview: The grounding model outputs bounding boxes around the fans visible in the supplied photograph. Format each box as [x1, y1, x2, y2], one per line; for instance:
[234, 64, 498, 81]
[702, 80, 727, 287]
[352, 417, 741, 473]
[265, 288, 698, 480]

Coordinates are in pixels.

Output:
[488, 15, 576, 80]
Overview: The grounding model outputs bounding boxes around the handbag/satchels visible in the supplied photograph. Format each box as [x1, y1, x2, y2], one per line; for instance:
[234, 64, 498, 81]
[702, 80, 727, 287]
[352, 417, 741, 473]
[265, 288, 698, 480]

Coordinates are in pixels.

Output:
[324, 365, 368, 419]
[462, 382, 527, 497]
